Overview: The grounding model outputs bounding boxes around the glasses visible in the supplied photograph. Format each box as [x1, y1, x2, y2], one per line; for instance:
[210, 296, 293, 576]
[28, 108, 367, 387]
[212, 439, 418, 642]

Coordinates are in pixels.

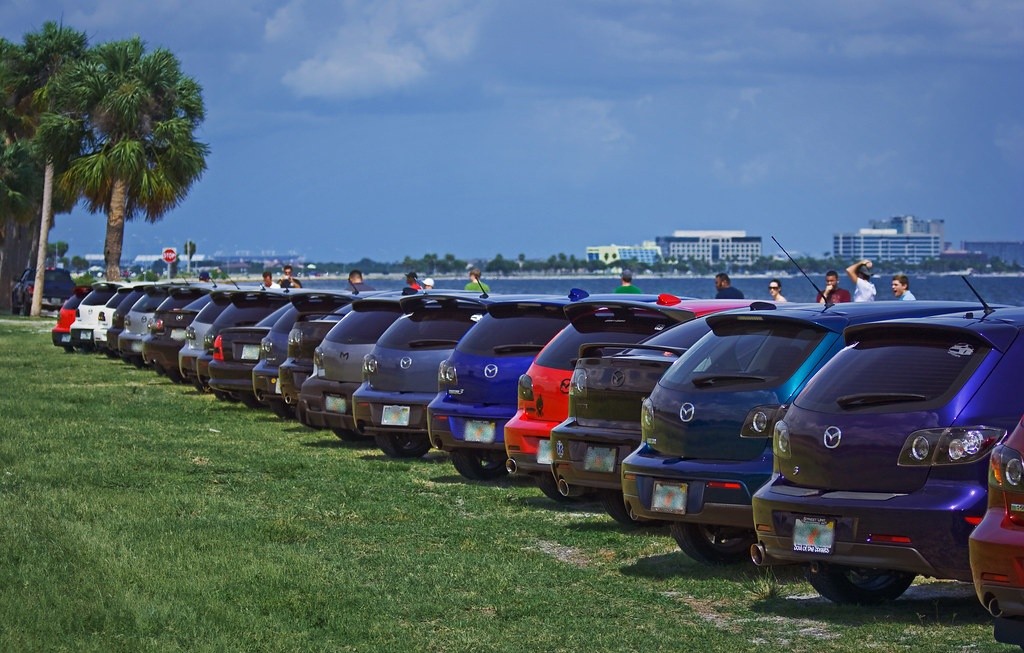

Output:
[892, 284, 898, 286]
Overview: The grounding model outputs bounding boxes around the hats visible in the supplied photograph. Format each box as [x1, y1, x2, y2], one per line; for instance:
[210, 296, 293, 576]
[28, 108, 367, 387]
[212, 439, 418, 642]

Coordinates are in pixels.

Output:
[853, 260, 874, 276]
[620, 270, 632, 281]
[768, 282, 779, 287]
[405, 272, 418, 280]
[422, 278, 434, 287]
[199, 271, 211, 280]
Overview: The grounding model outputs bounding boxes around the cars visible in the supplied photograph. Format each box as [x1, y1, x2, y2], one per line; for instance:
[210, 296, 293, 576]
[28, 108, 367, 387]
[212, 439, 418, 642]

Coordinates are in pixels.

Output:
[298, 293, 479, 445]
[253, 287, 391, 423]
[621, 300, 1006, 570]
[968, 413, 1024, 651]
[352, 292, 558, 461]
[426, 288, 696, 483]
[750, 304, 1024, 612]
[502, 298, 767, 504]
[279, 288, 460, 409]
[550, 302, 788, 528]
[52, 280, 267, 392]
[207, 302, 295, 411]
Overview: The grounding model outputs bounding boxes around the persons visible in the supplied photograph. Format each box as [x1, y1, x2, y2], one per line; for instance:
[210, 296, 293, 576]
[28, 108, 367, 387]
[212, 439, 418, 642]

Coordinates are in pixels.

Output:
[465, 268, 491, 292]
[404, 272, 434, 290]
[769, 279, 787, 302]
[198, 271, 211, 283]
[715, 273, 745, 299]
[846, 260, 877, 302]
[261, 265, 302, 288]
[816, 271, 851, 302]
[891, 275, 917, 301]
[344, 269, 376, 292]
[612, 269, 643, 293]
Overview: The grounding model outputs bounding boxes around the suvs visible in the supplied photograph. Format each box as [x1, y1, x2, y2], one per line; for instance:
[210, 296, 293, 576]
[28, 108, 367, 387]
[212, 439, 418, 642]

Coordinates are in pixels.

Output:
[11, 267, 76, 316]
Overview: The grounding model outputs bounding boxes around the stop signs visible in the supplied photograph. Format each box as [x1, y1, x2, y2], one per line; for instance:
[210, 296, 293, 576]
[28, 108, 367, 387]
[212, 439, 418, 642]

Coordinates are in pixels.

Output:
[161, 246, 176, 264]
